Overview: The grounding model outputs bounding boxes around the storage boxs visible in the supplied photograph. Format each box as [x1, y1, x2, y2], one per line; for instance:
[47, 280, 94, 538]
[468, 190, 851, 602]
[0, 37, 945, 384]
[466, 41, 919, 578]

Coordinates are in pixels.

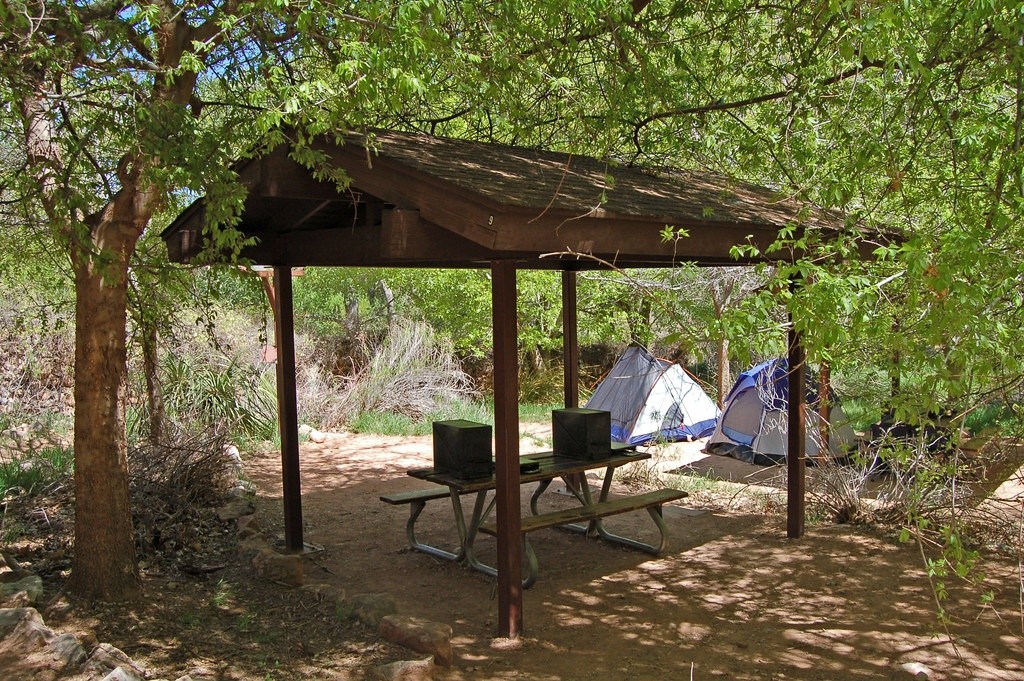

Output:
[552, 408, 611, 461]
[433, 420, 492, 481]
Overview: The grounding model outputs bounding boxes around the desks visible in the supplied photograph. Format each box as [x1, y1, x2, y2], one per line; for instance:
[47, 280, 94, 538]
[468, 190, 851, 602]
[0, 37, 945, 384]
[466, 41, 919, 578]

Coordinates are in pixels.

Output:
[406, 451, 652, 566]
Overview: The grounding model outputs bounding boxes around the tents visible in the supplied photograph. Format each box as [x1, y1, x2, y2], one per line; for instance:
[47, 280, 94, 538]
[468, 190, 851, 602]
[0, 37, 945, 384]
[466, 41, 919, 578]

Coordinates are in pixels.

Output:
[584, 344, 724, 446]
[700, 356, 862, 465]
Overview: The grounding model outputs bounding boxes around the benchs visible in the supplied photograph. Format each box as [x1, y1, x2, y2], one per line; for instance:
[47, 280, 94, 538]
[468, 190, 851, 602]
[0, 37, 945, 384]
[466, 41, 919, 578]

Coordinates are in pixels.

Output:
[478, 488, 688, 589]
[380, 478, 554, 548]
